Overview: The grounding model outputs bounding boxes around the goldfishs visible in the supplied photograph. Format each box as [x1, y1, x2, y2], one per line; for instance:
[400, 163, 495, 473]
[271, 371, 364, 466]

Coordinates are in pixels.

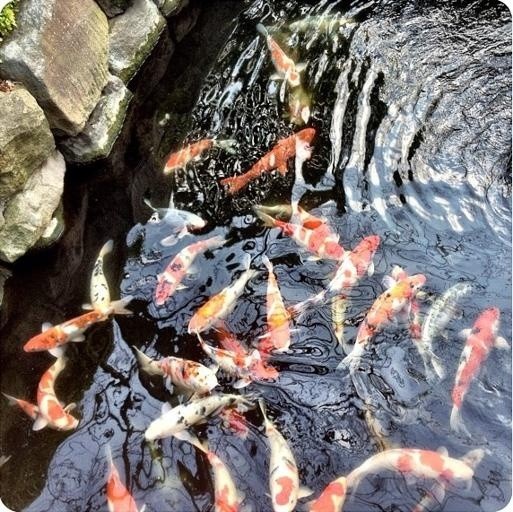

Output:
[102, 189, 511, 512]
